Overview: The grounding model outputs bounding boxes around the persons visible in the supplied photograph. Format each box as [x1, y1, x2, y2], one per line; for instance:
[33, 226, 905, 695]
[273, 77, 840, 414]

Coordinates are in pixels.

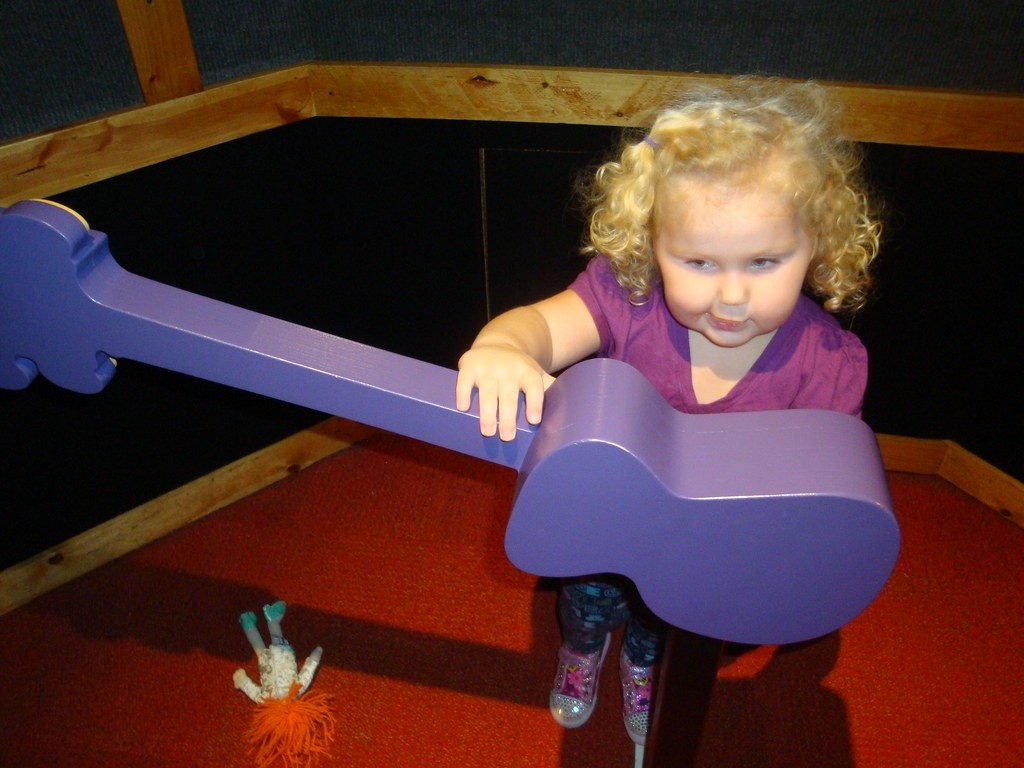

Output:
[455, 75, 881, 746]
[232, 600, 337, 768]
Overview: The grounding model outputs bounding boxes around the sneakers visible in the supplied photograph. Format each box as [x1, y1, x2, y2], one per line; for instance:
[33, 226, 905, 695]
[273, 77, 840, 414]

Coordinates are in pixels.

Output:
[619, 647, 654, 744]
[550, 630, 610, 727]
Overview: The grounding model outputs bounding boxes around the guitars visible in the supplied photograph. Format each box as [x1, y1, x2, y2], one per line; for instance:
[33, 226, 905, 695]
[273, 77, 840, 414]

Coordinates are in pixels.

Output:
[0, 192, 905, 648]
